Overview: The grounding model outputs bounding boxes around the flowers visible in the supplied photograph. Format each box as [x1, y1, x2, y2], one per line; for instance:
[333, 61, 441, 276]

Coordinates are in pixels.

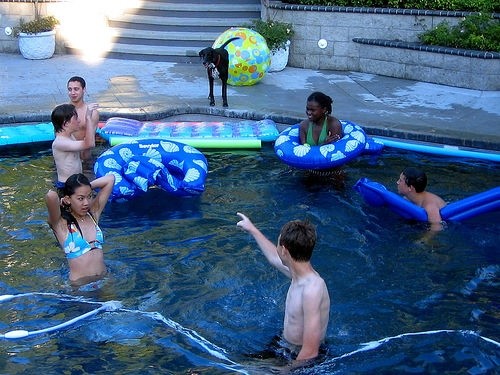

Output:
[249, 18, 296, 50]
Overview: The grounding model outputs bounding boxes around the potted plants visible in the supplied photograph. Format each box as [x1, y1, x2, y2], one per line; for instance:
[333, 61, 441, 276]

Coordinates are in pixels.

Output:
[11, 16, 60, 59]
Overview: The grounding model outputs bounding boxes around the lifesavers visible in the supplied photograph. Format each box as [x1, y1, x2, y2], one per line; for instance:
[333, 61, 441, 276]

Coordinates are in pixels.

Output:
[92, 138, 210, 201]
[109, 137, 261, 149]
[273, 118, 367, 171]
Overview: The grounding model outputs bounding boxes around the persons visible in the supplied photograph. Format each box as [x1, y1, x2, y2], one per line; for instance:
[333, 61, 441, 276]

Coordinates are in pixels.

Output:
[236, 212, 330, 359]
[46, 174, 115, 280]
[52, 103, 100, 190]
[61, 76, 99, 160]
[299, 92, 343, 180]
[396, 168, 446, 229]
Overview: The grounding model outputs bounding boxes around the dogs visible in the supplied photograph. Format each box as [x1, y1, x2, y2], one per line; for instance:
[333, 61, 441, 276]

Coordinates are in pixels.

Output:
[197, 37, 242, 107]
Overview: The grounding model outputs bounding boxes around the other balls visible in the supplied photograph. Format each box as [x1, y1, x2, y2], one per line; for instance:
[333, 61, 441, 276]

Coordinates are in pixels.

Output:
[212, 27, 270, 87]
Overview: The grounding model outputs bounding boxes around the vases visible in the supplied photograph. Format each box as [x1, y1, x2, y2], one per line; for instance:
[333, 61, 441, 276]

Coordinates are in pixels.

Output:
[267, 40, 291, 72]
